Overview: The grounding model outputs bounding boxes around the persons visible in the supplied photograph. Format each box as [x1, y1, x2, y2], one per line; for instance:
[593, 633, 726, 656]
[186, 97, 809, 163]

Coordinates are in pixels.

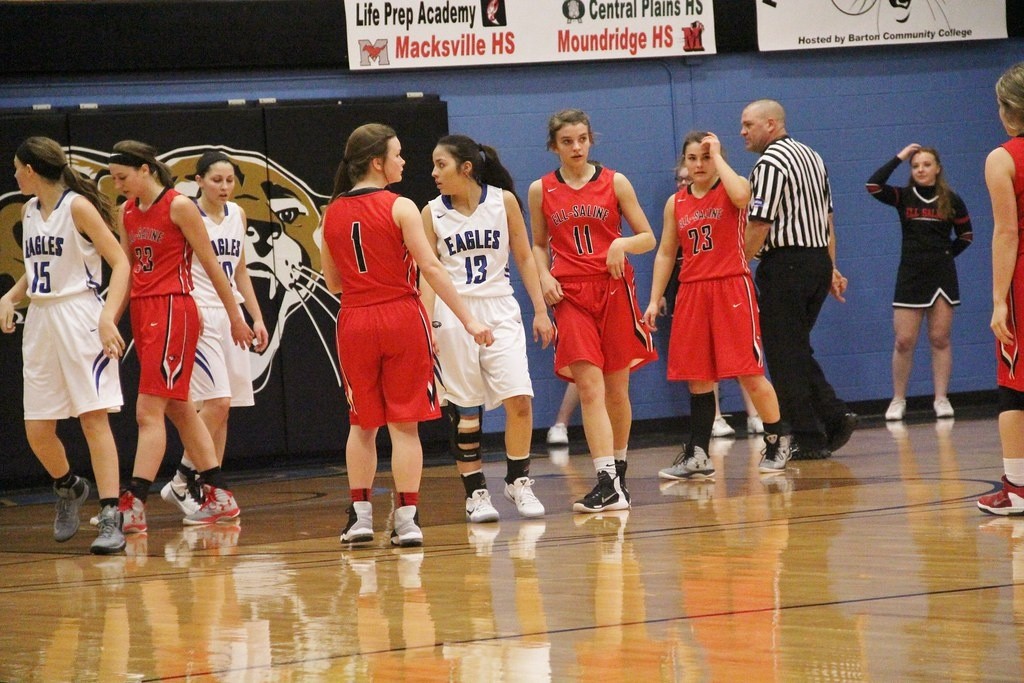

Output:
[0, 137, 130, 553]
[547, 381, 580, 443]
[320, 123, 494, 545]
[740, 99, 858, 459]
[420, 135, 554, 523]
[866, 143, 974, 419]
[160, 153, 268, 518]
[663, 163, 765, 436]
[977, 61, 1024, 515]
[90, 139, 255, 533]
[642, 130, 793, 482]
[528, 108, 656, 512]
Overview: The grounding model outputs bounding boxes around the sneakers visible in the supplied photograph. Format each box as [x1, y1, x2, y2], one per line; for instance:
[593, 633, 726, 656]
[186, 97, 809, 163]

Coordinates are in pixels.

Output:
[546, 423, 569, 443]
[977, 475, 1024, 515]
[54, 476, 89, 543]
[182, 484, 240, 525]
[90, 505, 126, 554]
[574, 460, 631, 512]
[829, 413, 857, 452]
[789, 433, 831, 459]
[934, 398, 954, 417]
[885, 398, 906, 419]
[340, 501, 376, 541]
[658, 442, 715, 479]
[161, 470, 204, 515]
[759, 432, 792, 473]
[504, 477, 545, 518]
[117, 489, 148, 532]
[747, 415, 764, 434]
[390, 504, 423, 547]
[710, 415, 735, 437]
[465, 488, 500, 522]
